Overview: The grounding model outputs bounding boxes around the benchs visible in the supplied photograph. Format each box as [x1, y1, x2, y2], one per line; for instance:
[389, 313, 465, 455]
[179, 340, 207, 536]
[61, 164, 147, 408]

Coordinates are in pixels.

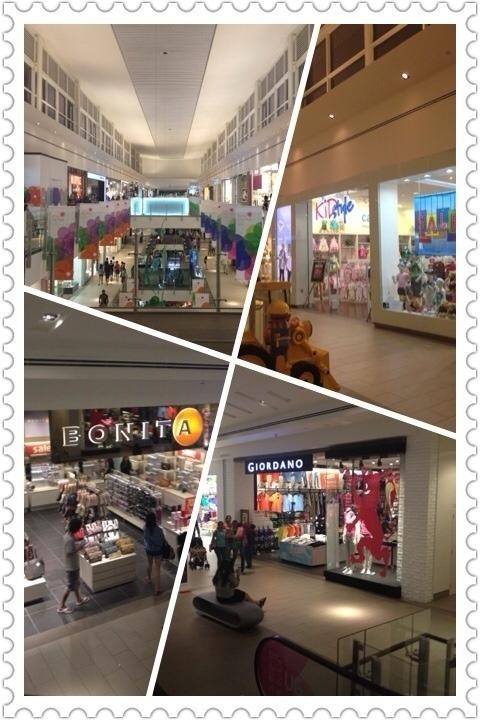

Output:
[193, 591, 264, 630]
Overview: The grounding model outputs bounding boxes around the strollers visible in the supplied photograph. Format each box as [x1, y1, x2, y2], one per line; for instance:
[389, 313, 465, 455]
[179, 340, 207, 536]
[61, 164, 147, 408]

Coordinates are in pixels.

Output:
[189, 537, 211, 571]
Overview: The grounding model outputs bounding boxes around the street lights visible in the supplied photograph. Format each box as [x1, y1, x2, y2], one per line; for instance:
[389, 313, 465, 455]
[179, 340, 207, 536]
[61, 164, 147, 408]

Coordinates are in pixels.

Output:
[128, 227, 145, 306]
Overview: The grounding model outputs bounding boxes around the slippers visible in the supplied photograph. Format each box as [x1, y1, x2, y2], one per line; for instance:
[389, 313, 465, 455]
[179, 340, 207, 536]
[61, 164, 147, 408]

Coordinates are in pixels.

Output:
[57, 597, 89, 615]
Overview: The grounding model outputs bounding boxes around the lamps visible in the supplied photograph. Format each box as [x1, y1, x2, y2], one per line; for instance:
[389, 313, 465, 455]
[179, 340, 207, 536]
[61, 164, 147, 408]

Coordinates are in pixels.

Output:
[339, 462, 343, 468]
[377, 458, 383, 467]
[359, 460, 364, 467]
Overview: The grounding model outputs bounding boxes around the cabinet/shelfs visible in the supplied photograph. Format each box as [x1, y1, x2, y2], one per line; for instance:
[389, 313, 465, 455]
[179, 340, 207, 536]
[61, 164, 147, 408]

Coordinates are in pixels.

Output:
[24, 450, 204, 568]
[312, 234, 370, 294]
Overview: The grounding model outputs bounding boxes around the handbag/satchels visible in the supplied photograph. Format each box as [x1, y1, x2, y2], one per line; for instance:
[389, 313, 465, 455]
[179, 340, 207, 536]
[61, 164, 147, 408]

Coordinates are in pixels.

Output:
[161, 462, 171, 470]
[24, 545, 45, 580]
[75, 511, 135, 563]
[163, 543, 176, 560]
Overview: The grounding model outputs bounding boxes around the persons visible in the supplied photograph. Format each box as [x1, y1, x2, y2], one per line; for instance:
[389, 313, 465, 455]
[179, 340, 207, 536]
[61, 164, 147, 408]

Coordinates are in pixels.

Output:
[444, 270, 456, 306]
[180, 231, 202, 252]
[238, 298, 342, 392]
[56, 518, 91, 614]
[119, 455, 134, 476]
[192, 539, 205, 564]
[278, 243, 287, 282]
[98, 256, 127, 286]
[393, 260, 412, 311]
[360, 542, 378, 577]
[101, 458, 115, 477]
[431, 278, 447, 313]
[142, 511, 168, 597]
[207, 241, 213, 256]
[208, 514, 256, 574]
[220, 253, 230, 275]
[98, 289, 109, 307]
[212, 557, 268, 609]
[263, 195, 270, 210]
[25, 456, 33, 482]
[342, 506, 358, 574]
[418, 269, 437, 313]
[285, 243, 292, 281]
[407, 262, 426, 312]
[204, 255, 208, 270]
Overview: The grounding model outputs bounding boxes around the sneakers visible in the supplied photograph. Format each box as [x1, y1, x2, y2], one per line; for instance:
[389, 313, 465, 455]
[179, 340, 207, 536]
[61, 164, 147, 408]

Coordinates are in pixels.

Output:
[259, 597, 266, 608]
